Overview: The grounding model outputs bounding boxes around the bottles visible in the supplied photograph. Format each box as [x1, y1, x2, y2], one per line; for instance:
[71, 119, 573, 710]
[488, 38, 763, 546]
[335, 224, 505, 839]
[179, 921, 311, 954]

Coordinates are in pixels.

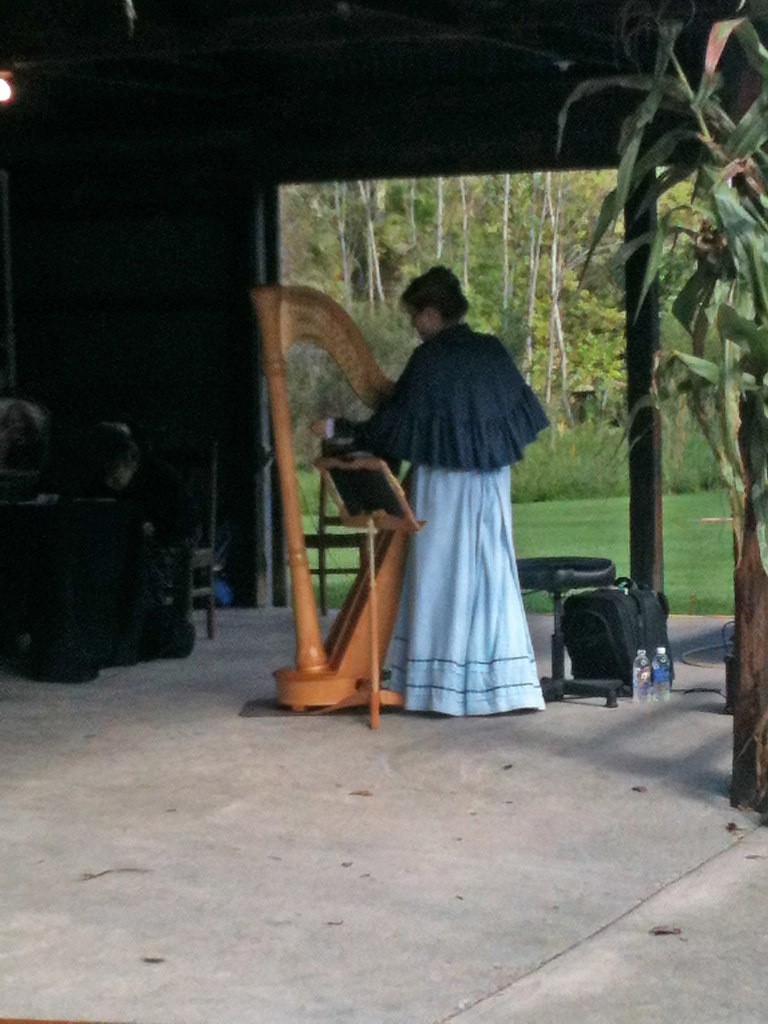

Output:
[631, 649, 653, 703]
[651, 647, 671, 702]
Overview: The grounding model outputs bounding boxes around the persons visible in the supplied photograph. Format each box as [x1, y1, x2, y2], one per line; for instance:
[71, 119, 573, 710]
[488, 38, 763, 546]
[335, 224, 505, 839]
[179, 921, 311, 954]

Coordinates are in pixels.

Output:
[308, 265, 554, 718]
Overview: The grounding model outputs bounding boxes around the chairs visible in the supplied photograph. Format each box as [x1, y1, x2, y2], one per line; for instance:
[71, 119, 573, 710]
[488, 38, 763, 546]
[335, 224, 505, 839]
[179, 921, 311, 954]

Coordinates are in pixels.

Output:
[166, 437, 216, 638]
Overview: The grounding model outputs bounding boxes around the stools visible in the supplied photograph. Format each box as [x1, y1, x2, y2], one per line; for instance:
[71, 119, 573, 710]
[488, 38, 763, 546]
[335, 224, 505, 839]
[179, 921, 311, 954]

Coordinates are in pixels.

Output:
[517, 556, 632, 707]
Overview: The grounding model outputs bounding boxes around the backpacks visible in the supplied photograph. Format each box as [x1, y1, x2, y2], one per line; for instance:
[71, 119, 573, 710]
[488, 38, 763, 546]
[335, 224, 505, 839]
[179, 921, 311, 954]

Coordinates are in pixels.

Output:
[563, 579, 672, 701]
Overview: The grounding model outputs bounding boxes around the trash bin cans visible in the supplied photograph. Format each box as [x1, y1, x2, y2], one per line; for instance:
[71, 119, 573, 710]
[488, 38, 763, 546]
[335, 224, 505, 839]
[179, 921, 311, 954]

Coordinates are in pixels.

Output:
[145, 541, 195, 631]
